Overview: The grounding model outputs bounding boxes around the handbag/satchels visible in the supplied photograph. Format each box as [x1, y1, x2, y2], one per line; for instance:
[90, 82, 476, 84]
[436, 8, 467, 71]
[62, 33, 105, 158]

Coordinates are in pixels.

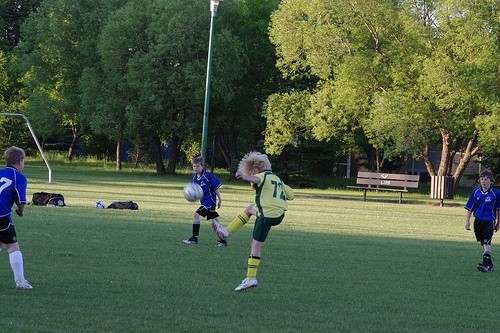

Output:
[48, 193, 66, 207]
[32, 192, 49, 206]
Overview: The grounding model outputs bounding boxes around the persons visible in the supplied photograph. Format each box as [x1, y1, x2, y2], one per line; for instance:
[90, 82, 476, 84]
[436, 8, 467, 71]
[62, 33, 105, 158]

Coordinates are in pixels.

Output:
[182, 157, 229, 247]
[211, 150, 294, 291]
[464, 169, 500, 274]
[0, 146, 33, 289]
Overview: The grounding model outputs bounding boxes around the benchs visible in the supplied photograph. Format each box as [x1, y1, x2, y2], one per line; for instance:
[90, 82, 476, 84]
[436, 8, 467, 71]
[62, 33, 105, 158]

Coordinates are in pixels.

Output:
[347, 172, 422, 204]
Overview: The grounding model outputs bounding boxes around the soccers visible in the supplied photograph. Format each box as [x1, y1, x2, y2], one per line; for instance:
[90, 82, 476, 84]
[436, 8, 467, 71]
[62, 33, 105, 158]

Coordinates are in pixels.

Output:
[183, 182, 204, 202]
[95, 200, 105, 208]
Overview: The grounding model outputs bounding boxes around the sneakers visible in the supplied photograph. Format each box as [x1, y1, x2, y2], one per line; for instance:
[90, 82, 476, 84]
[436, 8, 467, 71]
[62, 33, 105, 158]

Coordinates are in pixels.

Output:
[487, 266, 494, 273]
[182, 236, 198, 244]
[212, 219, 228, 239]
[16, 280, 33, 290]
[235, 277, 258, 292]
[477, 263, 488, 272]
[217, 240, 227, 247]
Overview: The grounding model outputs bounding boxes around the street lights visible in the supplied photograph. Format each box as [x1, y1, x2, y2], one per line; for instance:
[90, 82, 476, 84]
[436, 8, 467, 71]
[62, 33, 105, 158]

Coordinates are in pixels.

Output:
[200, 1, 221, 160]
